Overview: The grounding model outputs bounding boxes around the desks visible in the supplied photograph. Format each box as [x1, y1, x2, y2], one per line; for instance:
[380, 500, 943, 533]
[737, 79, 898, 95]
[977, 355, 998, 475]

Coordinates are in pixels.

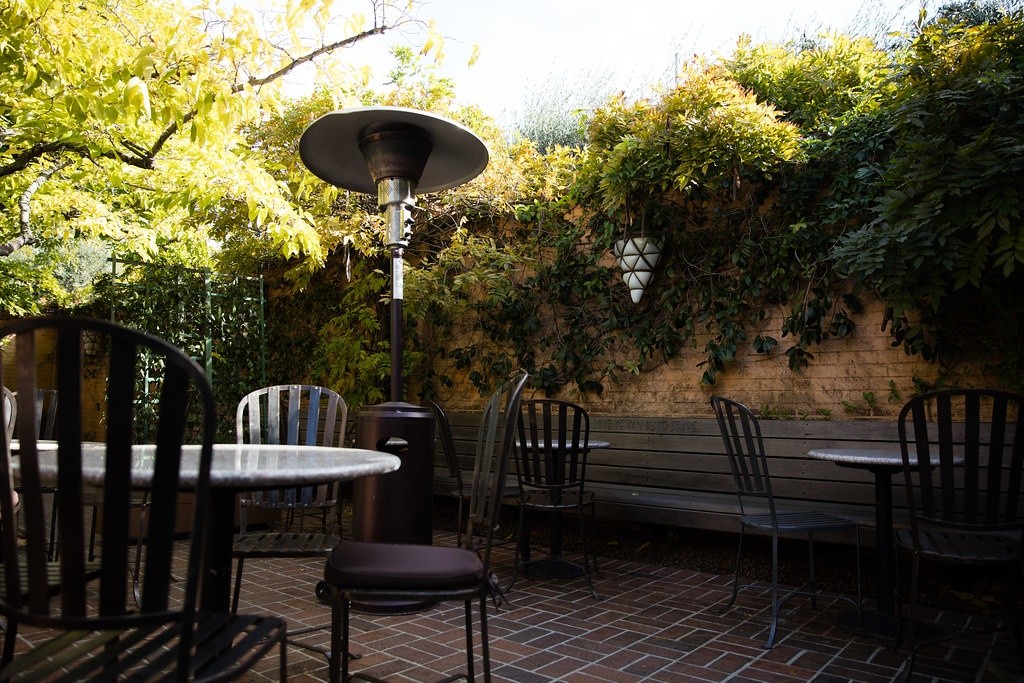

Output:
[10, 444, 402, 683]
[514, 438, 611, 580]
[807, 449, 965, 642]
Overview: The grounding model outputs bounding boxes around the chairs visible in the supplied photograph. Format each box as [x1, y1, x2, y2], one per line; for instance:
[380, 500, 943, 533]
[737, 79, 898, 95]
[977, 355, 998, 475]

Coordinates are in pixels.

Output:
[897, 389, 1024, 683]
[0, 314, 599, 683]
[710, 396, 865, 651]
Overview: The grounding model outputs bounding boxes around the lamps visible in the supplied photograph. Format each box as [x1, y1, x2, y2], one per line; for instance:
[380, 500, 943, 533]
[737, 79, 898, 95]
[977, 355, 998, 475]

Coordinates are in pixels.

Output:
[611, 222, 664, 304]
